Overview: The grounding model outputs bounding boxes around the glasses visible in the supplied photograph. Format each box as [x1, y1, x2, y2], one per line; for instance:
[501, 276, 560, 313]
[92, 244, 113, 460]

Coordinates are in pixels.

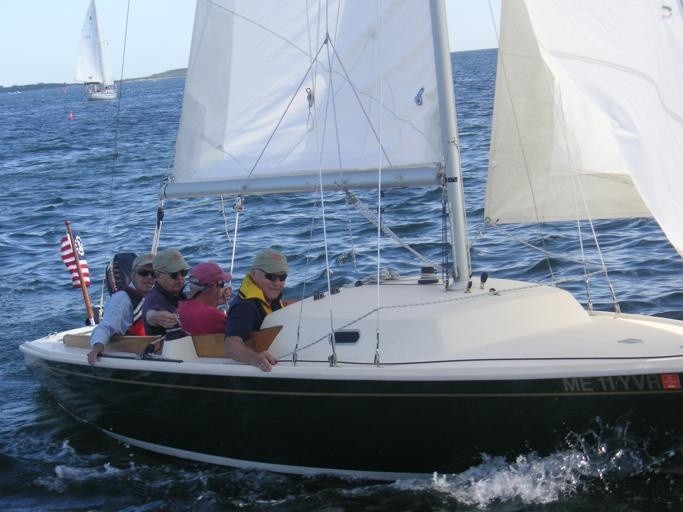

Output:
[257, 269, 288, 281]
[217, 282, 225, 288]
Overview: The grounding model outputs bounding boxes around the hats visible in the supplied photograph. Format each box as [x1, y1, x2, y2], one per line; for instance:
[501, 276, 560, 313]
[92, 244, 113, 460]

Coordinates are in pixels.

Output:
[132, 249, 232, 286]
[253, 249, 293, 273]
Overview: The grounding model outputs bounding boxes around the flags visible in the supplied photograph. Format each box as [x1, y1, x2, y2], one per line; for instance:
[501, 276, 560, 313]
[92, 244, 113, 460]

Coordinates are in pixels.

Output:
[59, 234, 91, 289]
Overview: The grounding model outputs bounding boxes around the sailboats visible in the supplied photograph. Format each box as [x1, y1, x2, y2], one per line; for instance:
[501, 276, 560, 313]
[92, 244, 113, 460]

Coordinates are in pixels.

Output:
[15, 0, 682, 499]
[73, 0, 119, 102]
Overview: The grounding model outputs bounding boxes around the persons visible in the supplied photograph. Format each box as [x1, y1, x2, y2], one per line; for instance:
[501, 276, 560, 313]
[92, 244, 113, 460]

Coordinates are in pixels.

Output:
[87, 249, 288, 372]
